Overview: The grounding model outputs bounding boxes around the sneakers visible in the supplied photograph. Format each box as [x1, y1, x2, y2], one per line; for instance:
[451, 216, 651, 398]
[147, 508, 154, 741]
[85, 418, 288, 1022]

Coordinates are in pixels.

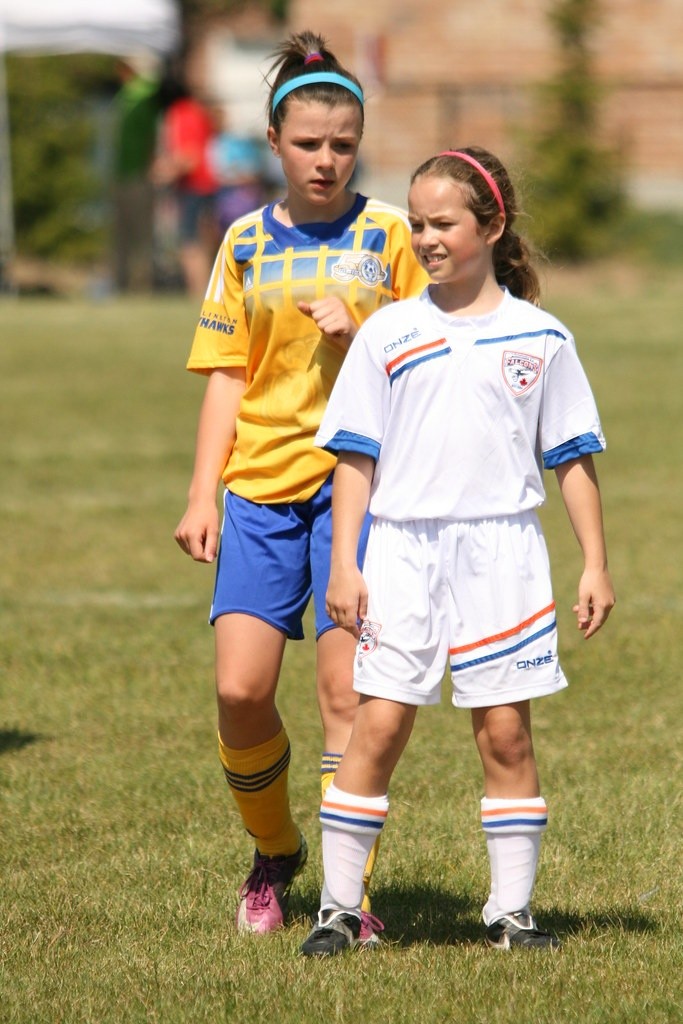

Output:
[235, 832, 309, 936]
[300, 905, 362, 961]
[358, 911, 384, 950]
[484, 910, 565, 956]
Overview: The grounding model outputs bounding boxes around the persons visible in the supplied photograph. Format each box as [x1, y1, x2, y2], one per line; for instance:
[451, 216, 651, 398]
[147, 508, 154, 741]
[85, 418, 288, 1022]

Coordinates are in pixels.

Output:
[176, 29, 438, 959]
[140, 65, 290, 299]
[296, 146, 620, 966]
